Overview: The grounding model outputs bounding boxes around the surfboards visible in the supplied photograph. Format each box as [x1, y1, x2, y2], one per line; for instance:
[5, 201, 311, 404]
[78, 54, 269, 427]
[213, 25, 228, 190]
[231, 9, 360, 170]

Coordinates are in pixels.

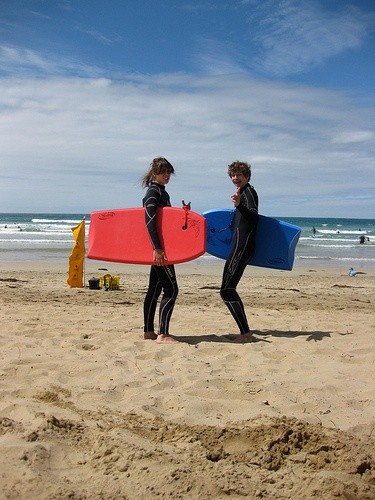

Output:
[199, 209, 301, 270]
[85, 209, 206, 266]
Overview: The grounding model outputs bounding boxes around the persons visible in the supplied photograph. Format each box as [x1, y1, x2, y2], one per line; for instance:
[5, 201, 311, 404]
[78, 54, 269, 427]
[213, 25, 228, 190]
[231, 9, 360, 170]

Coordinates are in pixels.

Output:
[359, 233, 366, 243]
[141, 157, 182, 344]
[337, 228, 340, 233]
[311, 226, 316, 237]
[221, 160, 259, 343]
